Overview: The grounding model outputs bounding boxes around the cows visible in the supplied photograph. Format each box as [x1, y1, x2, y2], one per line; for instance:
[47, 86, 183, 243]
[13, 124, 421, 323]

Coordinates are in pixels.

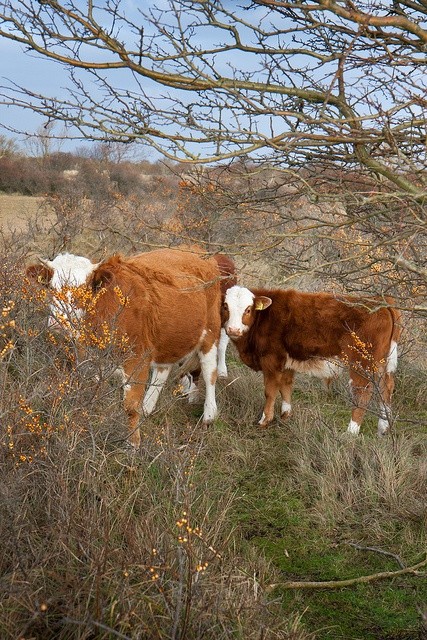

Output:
[303, 314, 307, 317]
[178, 252, 238, 404]
[221, 283, 403, 436]
[24, 245, 220, 451]
